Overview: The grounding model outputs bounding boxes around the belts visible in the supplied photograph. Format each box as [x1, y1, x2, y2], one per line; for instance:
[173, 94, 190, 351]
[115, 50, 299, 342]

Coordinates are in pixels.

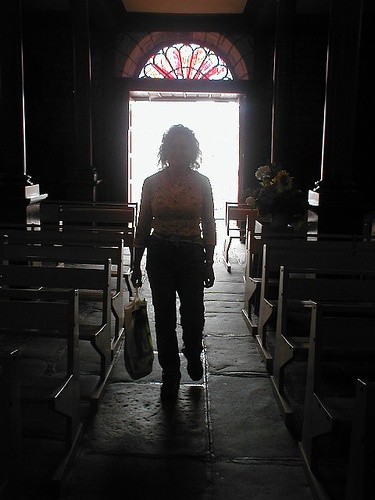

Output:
[152, 231, 208, 246]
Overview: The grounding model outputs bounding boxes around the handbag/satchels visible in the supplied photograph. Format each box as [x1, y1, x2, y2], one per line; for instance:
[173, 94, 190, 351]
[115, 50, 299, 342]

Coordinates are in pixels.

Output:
[124, 288, 154, 382]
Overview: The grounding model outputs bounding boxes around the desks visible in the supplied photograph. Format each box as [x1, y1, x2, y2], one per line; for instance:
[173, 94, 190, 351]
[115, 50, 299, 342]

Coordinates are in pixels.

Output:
[222, 202, 317, 276]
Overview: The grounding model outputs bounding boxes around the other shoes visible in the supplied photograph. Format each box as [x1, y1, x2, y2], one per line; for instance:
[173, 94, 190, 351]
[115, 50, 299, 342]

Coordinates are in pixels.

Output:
[187, 358, 204, 381]
[161, 376, 182, 397]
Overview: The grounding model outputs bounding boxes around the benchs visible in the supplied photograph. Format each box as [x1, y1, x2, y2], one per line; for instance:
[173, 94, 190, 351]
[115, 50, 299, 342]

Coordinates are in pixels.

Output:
[239, 230, 375, 337]
[0, 287, 86, 489]
[0, 258, 120, 407]
[255, 242, 375, 361]
[269, 265, 375, 414]
[0, 203, 143, 306]
[0, 238, 124, 357]
[298, 298, 375, 476]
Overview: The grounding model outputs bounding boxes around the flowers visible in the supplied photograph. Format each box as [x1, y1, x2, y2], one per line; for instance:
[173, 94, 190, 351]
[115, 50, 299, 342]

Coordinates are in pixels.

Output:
[245, 164, 296, 210]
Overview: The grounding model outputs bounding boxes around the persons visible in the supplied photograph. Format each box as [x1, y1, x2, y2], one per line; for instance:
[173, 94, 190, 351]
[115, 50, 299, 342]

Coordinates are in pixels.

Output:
[130, 124, 216, 405]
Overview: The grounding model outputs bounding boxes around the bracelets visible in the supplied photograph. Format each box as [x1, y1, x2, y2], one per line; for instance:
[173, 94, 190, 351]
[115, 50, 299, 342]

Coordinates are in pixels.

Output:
[205, 260, 214, 264]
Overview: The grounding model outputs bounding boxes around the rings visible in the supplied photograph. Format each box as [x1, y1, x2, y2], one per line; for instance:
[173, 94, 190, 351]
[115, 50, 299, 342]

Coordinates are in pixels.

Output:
[212, 277, 215, 279]
